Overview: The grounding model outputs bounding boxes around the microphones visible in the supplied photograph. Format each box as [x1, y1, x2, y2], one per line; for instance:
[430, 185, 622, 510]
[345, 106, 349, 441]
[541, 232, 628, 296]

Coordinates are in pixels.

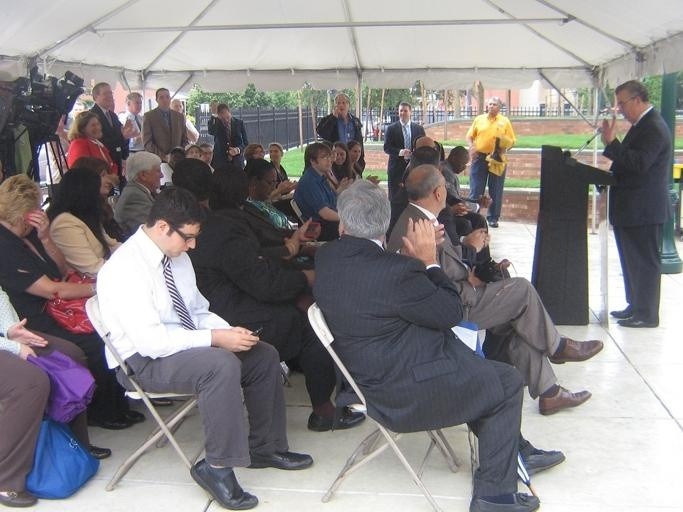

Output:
[571, 127, 604, 159]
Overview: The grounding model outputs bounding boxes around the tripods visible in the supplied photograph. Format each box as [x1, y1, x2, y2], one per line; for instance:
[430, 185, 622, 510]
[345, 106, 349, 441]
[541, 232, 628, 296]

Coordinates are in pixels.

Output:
[26, 133, 69, 184]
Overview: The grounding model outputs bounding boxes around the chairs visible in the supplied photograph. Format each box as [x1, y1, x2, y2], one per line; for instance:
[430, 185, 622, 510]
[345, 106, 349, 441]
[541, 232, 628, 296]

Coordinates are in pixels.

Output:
[84, 294, 201, 492]
[306, 300, 461, 512]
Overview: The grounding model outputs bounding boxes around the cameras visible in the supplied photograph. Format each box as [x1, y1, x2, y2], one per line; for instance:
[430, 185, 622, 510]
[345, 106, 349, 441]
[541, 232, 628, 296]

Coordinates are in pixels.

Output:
[11, 66, 85, 140]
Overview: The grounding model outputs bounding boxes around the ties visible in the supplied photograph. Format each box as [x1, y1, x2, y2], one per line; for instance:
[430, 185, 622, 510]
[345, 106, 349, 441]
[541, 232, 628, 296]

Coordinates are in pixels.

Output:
[106, 111, 113, 127]
[162, 255, 196, 330]
[404, 125, 410, 149]
[225, 122, 233, 145]
[135, 115, 141, 132]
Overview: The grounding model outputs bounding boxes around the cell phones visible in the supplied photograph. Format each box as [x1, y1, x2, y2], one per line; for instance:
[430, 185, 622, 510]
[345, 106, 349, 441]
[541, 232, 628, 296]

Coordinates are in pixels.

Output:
[250, 328, 263, 339]
[305, 222, 320, 237]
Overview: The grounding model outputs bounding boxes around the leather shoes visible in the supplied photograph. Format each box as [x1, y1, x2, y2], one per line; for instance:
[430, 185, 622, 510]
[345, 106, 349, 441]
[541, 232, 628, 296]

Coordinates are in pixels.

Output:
[468, 493, 540, 512]
[307, 405, 365, 432]
[190, 458, 259, 511]
[489, 219, 498, 228]
[538, 385, 592, 415]
[610, 305, 633, 319]
[0, 490, 37, 508]
[148, 398, 174, 406]
[247, 450, 314, 471]
[616, 315, 660, 328]
[114, 410, 146, 423]
[87, 413, 134, 430]
[517, 450, 566, 480]
[86, 444, 112, 460]
[548, 338, 604, 364]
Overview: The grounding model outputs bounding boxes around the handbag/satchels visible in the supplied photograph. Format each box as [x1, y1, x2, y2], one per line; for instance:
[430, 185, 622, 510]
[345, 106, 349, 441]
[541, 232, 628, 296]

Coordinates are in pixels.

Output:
[47, 271, 97, 334]
[24, 419, 99, 501]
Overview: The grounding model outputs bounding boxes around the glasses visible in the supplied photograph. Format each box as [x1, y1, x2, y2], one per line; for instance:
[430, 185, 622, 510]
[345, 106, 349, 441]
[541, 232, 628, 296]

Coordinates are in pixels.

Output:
[164, 220, 202, 242]
[315, 157, 332, 160]
[619, 96, 636, 108]
[262, 177, 278, 188]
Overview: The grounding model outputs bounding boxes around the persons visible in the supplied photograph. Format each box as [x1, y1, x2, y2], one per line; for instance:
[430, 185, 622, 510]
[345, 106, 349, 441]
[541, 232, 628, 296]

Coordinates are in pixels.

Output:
[321, 141, 354, 193]
[46, 113, 71, 207]
[347, 140, 382, 186]
[246, 144, 299, 221]
[0, 173, 146, 430]
[243, 158, 322, 241]
[317, 93, 366, 180]
[94, 187, 313, 512]
[170, 98, 199, 150]
[185, 145, 203, 160]
[87, 83, 139, 163]
[313, 180, 565, 512]
[268, 143, 298, 202]
[171, 157, 367, 432]
[331, 142, 359, 187]
[144, 87, 189, 162]
[66, 111, 119, 195]
[404, 137, 491, 262]
[119, 93, 144, 152]
[45, 168, 122, 281]
[293, 143, 340, 240]
[114, 152, 164, 237]
[208, 104, 249, 172]
[160, 147, 184, 186]
[209, 163, 318, 289]
[441, 145, 494, 213]
[1, 287, 111, 507]
[384, 103, 426, 203]
[391, 146, 512, 366]
[466, 97, 516, 227]
[440, 146, 473, 219]
[600, 80, 673, 328]
[201, 143, 216, 172]
[74, 156, 125, 245]
[387, 164, 604, 416]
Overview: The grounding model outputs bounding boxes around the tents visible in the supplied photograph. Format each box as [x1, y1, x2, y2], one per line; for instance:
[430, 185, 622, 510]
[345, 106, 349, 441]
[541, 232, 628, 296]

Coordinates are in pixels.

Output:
[1, 3, 683, 236]
